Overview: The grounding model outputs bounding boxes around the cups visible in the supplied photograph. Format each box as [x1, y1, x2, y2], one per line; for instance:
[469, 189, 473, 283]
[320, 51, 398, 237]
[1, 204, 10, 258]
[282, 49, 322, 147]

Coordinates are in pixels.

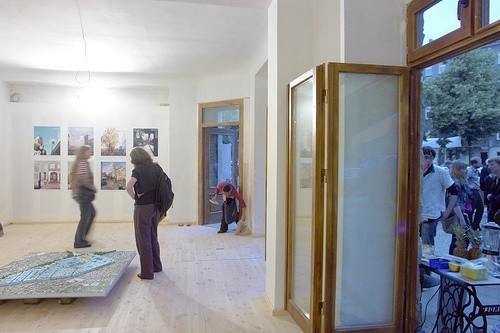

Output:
[448, 262, 460, 271]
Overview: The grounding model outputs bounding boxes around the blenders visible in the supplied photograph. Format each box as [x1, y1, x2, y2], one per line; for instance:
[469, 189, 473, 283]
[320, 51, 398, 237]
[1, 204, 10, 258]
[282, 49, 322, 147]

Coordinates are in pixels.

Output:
[479, 221, 500, 280]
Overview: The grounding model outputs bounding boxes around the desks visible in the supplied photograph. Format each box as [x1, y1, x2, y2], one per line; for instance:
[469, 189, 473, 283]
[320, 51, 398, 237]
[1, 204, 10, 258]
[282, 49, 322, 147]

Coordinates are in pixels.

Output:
[418, 245, 500, 333]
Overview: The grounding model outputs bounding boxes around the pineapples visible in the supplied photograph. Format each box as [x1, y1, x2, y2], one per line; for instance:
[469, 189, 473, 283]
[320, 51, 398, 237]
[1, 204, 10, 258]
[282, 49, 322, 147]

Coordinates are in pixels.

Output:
[451, 222, 483, 260]
[448, 262, 460, 272]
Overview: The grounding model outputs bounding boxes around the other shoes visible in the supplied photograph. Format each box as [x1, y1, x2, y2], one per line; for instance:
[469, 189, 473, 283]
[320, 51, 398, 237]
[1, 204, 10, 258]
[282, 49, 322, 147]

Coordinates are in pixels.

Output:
[217, 230, 226, 233]
[137, 273, 141, 277]
[79, 242, 91, 248]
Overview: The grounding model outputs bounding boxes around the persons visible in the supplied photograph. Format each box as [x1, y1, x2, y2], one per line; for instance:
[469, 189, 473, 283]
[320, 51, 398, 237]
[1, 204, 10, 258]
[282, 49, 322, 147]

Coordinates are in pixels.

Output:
[418, 146, 459, 276]
[467, 157, 484, 232]
[480, 157, 500, 228]
[126, 148, 163, 280]
[212, 181, 246, 233]
[70, 145, 97, 248]
[445, 160, 473, 255]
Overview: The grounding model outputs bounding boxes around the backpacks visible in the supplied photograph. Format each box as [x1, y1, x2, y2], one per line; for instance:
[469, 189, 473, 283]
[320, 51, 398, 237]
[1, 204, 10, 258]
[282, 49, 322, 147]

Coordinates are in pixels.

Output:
[155, 163, 174, 212]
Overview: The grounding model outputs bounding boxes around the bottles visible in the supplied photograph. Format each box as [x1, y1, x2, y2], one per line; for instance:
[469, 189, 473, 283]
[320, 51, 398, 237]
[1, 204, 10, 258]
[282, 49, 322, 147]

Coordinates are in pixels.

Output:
[418, 232, 422, 264]
[455, 260, 461, 272]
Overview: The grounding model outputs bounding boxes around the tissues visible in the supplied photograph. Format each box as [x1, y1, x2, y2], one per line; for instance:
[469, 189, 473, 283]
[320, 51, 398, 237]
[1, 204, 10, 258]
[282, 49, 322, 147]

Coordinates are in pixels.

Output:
[461, 260, 488, 281]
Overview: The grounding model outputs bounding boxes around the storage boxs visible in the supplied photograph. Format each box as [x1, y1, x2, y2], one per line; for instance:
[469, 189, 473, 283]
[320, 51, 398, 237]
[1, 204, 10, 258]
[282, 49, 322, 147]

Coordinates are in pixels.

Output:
[428, 258, 488, 280]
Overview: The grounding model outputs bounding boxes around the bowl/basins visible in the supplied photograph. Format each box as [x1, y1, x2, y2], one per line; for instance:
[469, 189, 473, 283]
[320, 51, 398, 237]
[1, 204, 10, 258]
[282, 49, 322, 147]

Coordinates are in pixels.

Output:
[428, 258, 450, 269]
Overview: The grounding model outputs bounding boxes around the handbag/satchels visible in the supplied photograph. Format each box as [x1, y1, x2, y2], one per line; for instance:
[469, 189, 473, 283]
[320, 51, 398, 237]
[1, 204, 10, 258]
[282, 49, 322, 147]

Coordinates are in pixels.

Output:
[77, 186, 95, 202]
[224, 203, 237, 224]
[441, 213, 458, 234]
